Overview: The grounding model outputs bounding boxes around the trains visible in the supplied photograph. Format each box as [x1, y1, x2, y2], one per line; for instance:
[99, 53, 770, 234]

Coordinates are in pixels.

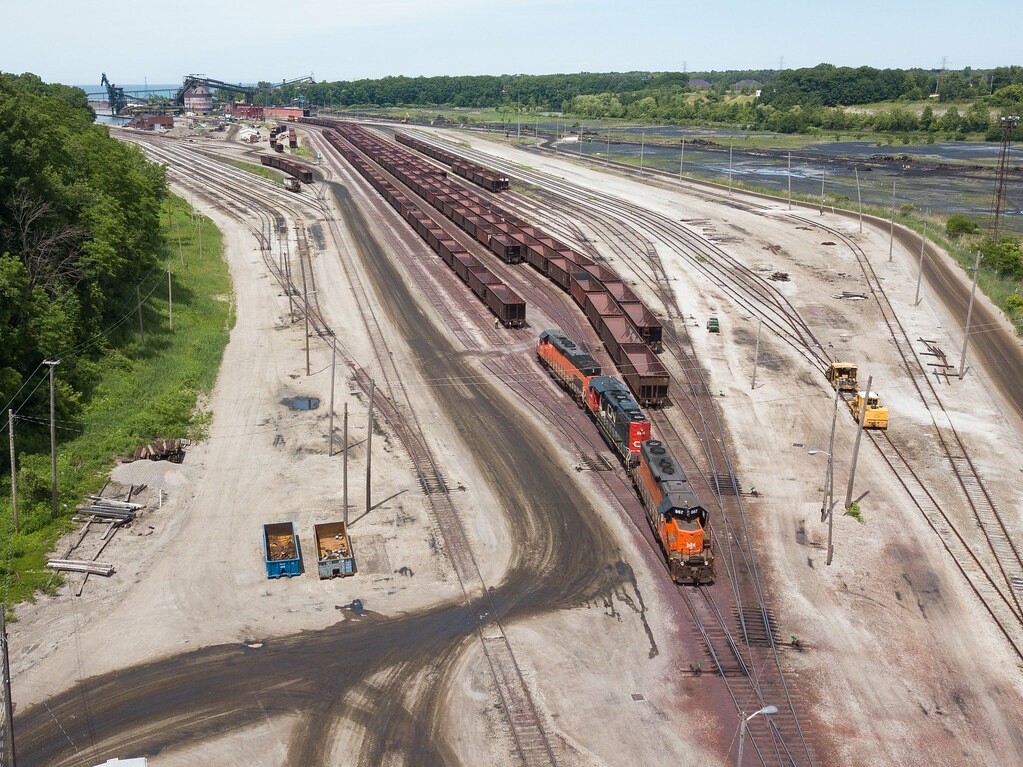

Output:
[826, 360, 890, 430]
[536, 328, 717, 588]
